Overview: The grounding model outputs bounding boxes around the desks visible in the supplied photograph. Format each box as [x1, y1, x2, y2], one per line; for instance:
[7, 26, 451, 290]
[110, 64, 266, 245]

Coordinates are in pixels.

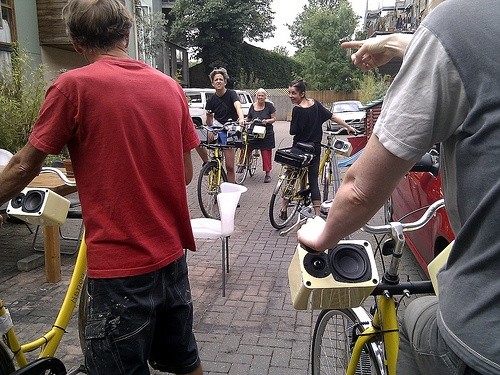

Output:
[26, 168, 78, 283]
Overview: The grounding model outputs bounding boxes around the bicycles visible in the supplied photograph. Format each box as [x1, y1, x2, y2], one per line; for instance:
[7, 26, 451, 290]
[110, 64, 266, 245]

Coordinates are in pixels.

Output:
[279, 198, 455, 375]
[0, 166, 88, 375]
[234, 118, 260, 185]
[194, 119, 249, 218]
[269, 127, 354, 230]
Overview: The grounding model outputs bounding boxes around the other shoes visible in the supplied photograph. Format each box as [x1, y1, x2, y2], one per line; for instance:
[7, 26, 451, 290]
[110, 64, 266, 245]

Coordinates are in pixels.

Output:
[200, 161, 212, 175]
[278, 208, 287, 220]
[236, 165, 246, 174]
[264, 175, 271, 183]
[236, 203, 240, 207]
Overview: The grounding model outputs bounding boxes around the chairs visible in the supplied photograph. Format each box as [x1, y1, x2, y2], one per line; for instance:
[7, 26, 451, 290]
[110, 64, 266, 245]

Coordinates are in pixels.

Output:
[183, 181, 248, 298]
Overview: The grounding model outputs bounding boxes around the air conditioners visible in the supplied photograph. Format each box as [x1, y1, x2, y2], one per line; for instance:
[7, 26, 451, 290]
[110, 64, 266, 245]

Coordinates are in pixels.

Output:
[136, 5, 151, 19]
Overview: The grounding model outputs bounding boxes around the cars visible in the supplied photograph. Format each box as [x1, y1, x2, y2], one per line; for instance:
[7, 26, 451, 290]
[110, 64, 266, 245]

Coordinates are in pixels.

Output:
[327, 101, 367, 132]
[384, 142, 455, 281]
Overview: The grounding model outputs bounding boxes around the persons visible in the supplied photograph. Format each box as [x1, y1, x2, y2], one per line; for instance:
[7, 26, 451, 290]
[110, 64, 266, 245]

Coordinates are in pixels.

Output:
[297, 0, 500, 375]
[195, 68, 245, 183]
[279, 79, 356, 220]
[236, 88, 276, 182]
[0, 0, 204, 375]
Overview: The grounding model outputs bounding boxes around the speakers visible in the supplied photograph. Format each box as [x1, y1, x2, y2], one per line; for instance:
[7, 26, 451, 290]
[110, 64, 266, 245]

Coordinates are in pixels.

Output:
[252, 124, 267, 139]
[6, 186, 70, 227]
[332, 139, 353, 158]
[288, 239, 380, 308]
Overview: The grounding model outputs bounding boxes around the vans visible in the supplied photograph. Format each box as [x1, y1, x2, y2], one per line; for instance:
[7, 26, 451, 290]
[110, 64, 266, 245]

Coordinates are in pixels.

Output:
[182, 87, 254, 129]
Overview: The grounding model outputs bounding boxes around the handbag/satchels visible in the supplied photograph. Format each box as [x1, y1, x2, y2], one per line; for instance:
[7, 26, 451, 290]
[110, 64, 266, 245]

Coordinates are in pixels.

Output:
[274, 146, 317, 169]
